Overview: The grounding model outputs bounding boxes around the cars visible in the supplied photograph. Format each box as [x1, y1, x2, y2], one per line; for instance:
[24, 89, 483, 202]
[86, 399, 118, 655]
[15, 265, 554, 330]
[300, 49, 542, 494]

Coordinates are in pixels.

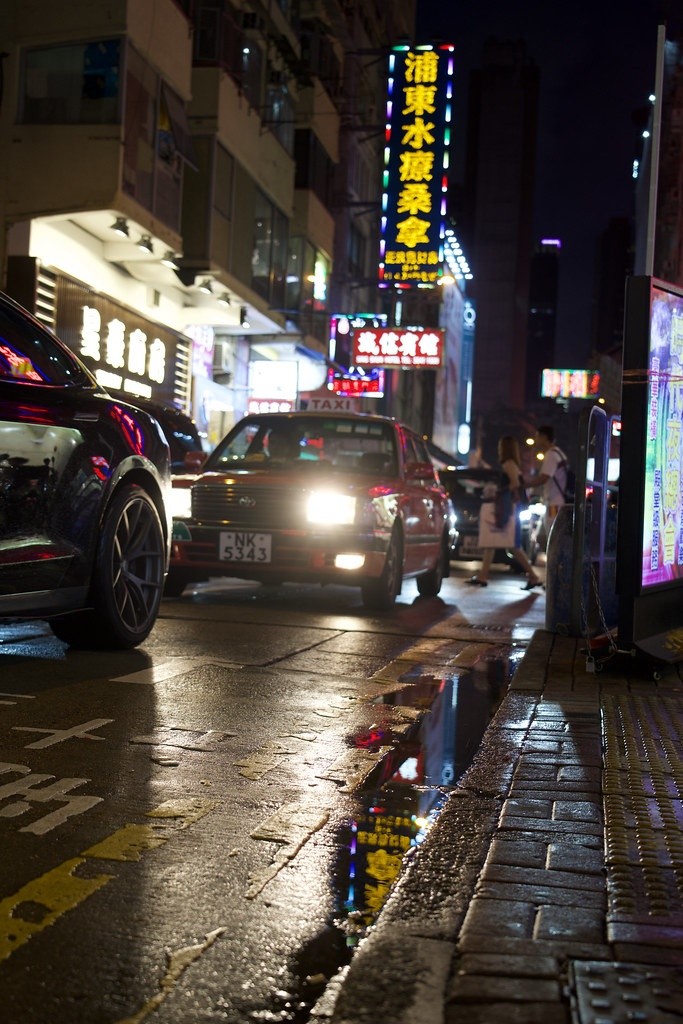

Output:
[0, 291, 172, 653]
[434, 463, 532, 572]
[169, 408, 454, 615]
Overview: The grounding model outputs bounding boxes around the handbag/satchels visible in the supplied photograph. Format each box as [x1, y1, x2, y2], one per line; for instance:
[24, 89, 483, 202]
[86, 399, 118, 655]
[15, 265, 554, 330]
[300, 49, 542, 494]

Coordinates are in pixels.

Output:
[477, 502, 516, 549]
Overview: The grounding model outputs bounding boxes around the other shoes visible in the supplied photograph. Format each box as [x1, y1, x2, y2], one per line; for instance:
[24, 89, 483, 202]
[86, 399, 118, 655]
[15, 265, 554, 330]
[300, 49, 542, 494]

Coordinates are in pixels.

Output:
[464, 575, 488, 587]
[521, 579, 542, 590]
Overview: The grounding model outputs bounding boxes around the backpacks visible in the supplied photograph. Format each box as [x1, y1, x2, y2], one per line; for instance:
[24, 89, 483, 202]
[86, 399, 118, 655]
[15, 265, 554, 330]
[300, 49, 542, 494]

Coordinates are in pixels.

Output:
[550, 448, 575, 503]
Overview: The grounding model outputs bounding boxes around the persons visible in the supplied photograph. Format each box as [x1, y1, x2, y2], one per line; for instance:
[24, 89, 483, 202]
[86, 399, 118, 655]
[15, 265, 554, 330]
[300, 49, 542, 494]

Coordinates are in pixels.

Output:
[495, 434, 532, 577]
[465, 434, 543, 590]
[515, 426, 571, 590]
[268, 428, 298, 458]
